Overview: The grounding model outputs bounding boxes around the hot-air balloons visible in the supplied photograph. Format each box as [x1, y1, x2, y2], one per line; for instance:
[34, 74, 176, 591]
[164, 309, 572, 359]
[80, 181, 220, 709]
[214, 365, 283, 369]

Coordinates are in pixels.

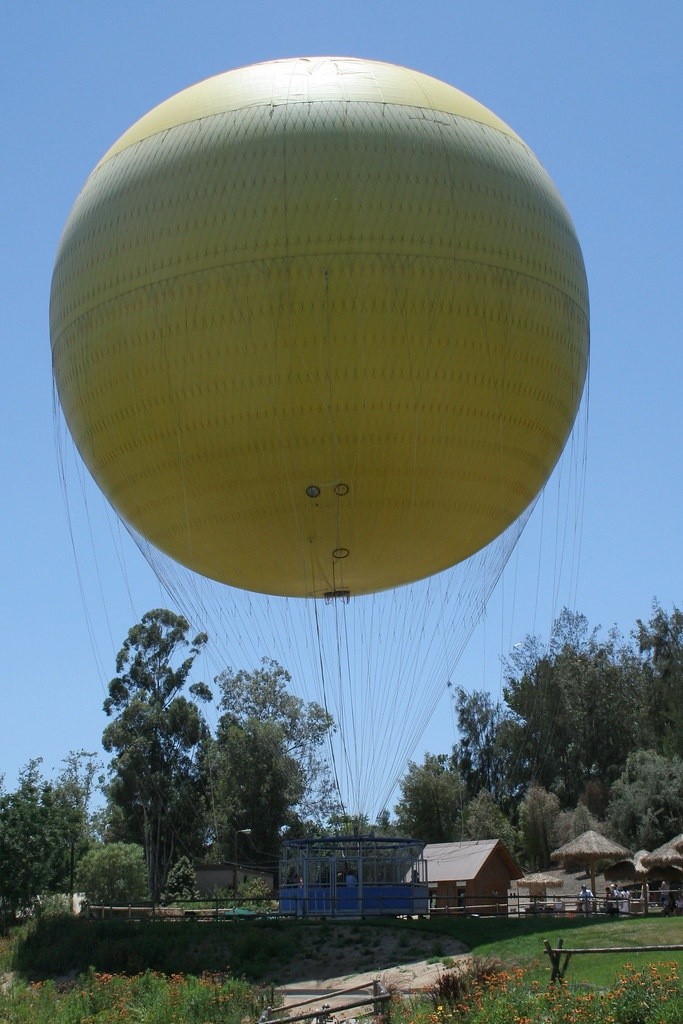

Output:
[48, 51, 591, 934]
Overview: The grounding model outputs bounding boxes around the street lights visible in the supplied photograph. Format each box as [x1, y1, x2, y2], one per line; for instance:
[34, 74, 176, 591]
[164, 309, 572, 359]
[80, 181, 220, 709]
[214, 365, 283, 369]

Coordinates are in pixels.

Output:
[232, 828, 252, 910]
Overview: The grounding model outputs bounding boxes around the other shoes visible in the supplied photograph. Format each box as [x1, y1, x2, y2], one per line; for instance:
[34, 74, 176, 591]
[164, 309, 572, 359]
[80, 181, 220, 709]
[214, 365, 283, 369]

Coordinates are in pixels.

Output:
[655, 904, 658, 906]
[651, 904, 654, 907]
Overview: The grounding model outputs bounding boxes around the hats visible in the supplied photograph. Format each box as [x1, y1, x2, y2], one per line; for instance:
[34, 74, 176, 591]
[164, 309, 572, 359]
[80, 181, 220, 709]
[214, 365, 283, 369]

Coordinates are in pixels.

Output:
[611, 883, 616, 888]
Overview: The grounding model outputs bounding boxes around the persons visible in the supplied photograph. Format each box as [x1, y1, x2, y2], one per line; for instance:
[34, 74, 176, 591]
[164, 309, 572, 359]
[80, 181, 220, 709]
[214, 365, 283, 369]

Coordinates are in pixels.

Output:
[345, 871, 357, 887]
[579, 884, 594, 911]
[659, 879, 672, 896]
[605, 883, 631, 899]
[662, 892, 675, 917]
[639, 883, 650, 904]
[287, 866, 300, 888]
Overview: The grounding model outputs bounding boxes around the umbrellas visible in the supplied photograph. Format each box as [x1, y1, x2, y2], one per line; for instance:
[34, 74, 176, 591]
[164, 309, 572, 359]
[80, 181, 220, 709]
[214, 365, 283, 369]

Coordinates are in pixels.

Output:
[604, 848, 677, 914]
[641, 833, 683, 866]
[517, 872, 562, 889]
[551, 829, 633, 897]
